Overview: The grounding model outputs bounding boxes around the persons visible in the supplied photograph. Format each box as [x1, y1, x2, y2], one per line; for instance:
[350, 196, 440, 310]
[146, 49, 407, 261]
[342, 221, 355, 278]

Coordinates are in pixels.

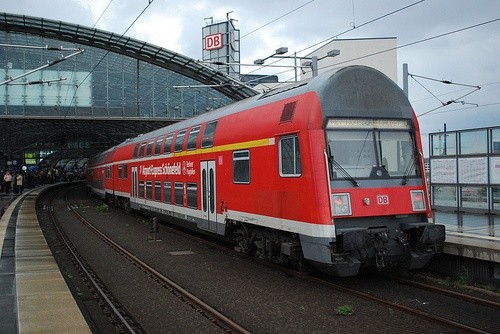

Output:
[1, 164, 89, 197]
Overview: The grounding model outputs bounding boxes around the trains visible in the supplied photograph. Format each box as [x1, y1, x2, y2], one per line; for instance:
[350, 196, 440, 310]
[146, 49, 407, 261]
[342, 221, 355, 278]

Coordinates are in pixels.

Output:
[86, 63, 446, 278]
[79, 174, 134, 198]
